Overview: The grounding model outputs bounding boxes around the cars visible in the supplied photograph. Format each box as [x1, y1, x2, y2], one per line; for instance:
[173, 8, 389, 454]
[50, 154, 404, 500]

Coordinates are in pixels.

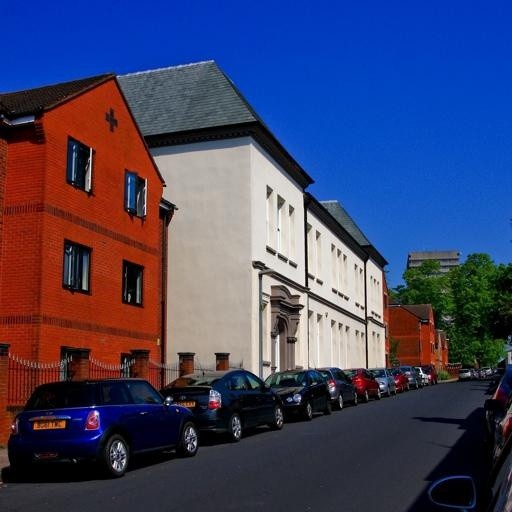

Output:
[7, 377, 199, 477]
[316, 367, 358, 409]
[485, 365, 512, 434]
[428, 404, 511, 512]
[265, 369, 332, 421]
[158, 368, 285, 443]
[343, 365, 439, 402]
[457, 365, 501, 383]
